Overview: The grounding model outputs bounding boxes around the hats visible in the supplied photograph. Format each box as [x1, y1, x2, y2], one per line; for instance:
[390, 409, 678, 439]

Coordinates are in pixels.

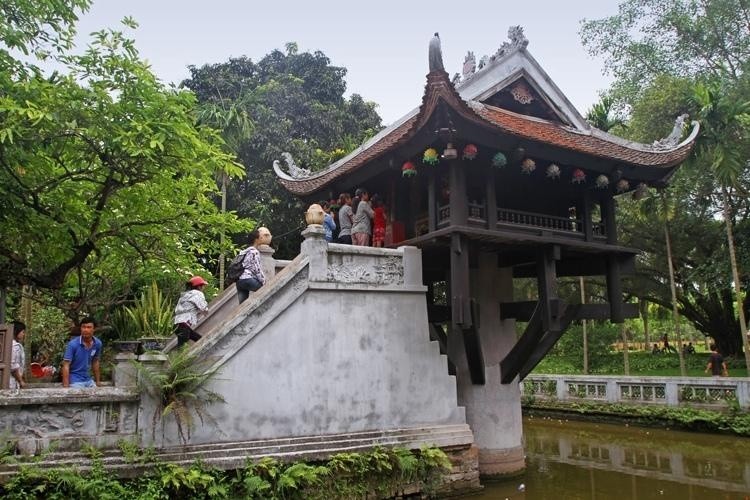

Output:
[189, 276, 210, 287]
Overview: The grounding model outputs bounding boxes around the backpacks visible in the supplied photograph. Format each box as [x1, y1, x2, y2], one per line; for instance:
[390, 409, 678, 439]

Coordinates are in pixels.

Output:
[228, 254, 246, 281]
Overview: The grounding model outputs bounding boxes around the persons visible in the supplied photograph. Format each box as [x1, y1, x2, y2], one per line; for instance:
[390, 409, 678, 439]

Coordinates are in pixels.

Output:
[705, 344, 729, 377]
[652, 334, 697, 356]
[173, 276, 209, 352]
[8, 320, 29, 389]
[236, 230, 267, 305]
[62, 317, 103, 388]
[318, 187, 388, 247]
